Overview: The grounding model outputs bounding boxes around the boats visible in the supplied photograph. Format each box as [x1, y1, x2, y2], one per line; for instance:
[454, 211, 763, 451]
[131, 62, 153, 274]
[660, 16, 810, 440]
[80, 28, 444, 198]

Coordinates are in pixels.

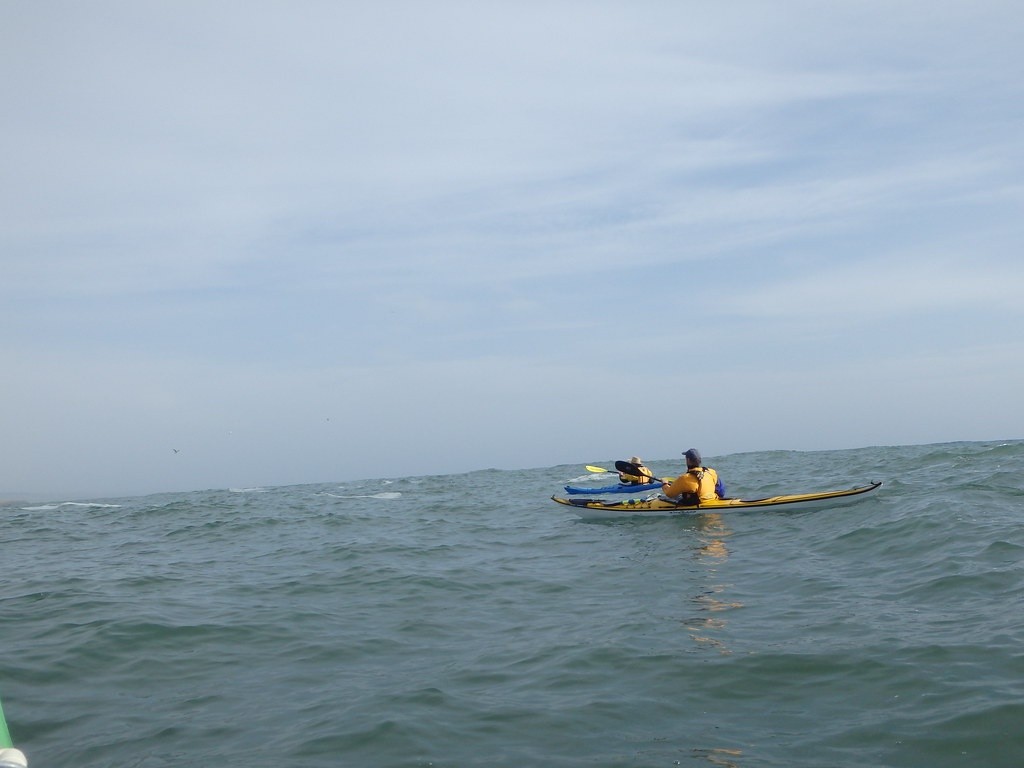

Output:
[563, 482, 670, 494]
[551, 481, 884, 511]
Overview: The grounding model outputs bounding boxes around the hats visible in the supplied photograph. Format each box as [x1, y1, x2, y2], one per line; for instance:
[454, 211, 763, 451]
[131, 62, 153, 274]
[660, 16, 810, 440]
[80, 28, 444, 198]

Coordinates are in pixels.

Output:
[682, 448, 700, 458]
[628, 457, 643, 464]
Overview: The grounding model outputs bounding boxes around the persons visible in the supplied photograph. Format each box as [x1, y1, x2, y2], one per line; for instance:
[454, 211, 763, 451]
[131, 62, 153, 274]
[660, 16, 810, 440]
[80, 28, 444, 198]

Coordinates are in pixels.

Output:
[619, 457, 656, 485]
[660, 448, 725, 506]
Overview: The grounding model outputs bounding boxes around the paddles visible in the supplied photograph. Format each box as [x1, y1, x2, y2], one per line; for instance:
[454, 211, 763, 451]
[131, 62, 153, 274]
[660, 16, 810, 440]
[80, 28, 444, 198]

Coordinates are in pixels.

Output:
[585, 464, 676, 482]
[615, 459, 672, 487]
